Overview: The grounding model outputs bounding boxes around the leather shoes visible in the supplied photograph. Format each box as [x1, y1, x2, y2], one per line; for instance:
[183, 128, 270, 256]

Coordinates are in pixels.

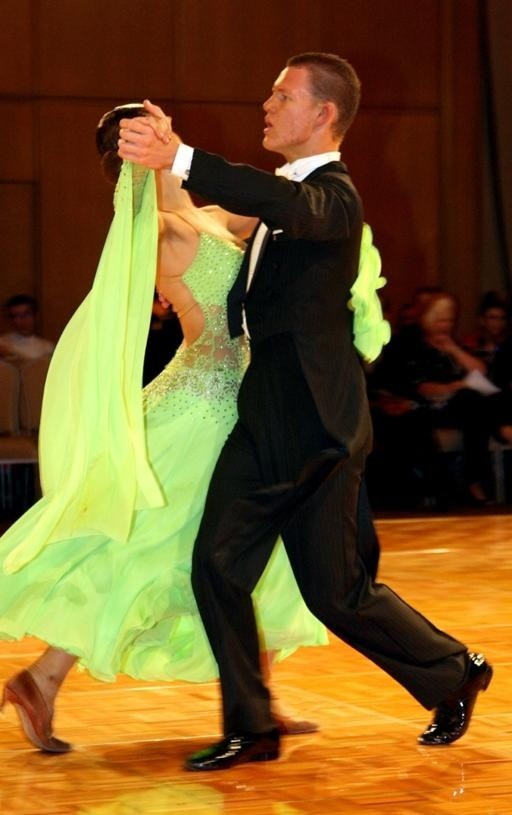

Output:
[186, 731, 281, 771]
[417, 652, 493, 745]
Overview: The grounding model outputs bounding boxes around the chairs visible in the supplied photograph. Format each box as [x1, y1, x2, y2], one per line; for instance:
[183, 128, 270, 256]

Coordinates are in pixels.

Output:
[22, 354, 51, 440]
[489, 439, 511, 507]
[0, 360, 36, 480]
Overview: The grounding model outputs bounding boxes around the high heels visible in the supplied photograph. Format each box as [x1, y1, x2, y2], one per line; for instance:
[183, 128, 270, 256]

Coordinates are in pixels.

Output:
[0, 669, 72, 752]
[271, 703, 320, 734]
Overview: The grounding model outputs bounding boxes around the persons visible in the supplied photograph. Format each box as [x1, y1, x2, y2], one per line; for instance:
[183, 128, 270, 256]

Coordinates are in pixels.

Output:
[140, 285, 511, 520]
[0, 292, 59, 519]
[0, 99, 399, 755]
[114, 45, 499, 777]
